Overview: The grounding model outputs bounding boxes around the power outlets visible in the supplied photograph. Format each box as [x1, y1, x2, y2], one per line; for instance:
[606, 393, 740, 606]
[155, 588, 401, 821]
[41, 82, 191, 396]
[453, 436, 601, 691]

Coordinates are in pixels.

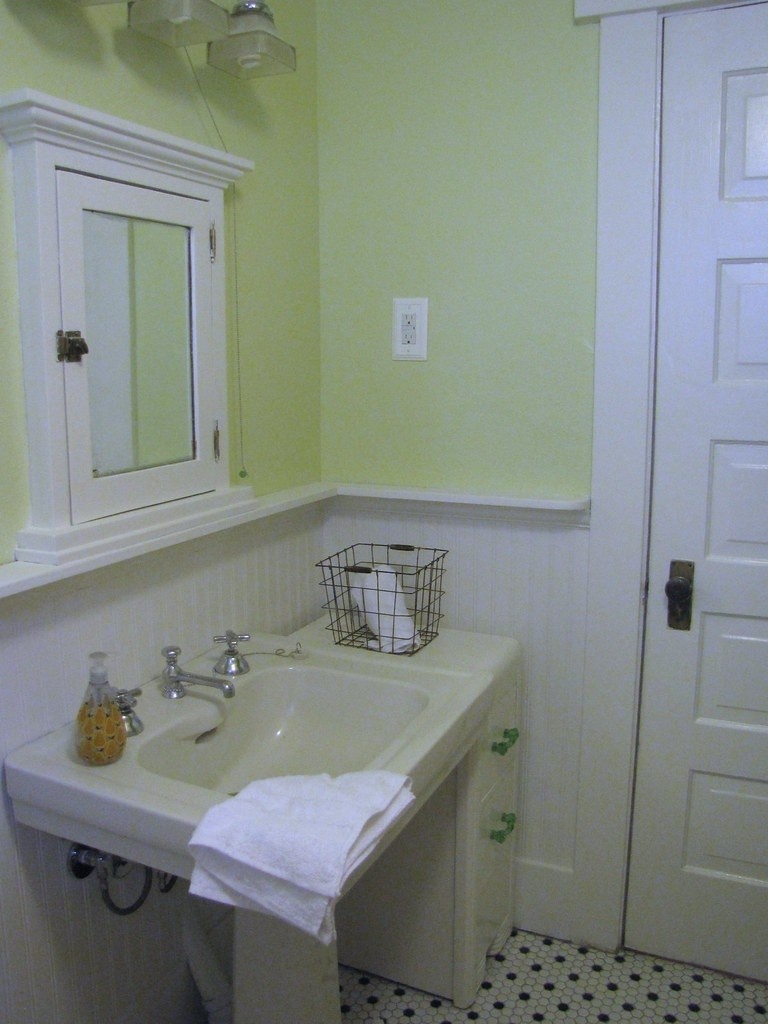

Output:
[394, 297, 428, 361]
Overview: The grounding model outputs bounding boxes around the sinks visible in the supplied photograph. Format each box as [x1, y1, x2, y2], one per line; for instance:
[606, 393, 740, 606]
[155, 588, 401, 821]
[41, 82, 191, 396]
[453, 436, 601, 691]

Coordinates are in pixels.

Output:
[1, 638, 500, 919]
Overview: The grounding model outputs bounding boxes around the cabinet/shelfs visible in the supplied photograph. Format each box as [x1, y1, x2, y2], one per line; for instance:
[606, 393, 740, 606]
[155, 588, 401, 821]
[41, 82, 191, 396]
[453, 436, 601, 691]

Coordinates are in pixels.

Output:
[332, 683, 525, 1009]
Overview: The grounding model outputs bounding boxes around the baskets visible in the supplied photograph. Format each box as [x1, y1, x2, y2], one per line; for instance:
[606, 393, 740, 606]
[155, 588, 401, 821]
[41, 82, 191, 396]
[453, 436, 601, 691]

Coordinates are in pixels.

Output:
[316, 543, 449, 657]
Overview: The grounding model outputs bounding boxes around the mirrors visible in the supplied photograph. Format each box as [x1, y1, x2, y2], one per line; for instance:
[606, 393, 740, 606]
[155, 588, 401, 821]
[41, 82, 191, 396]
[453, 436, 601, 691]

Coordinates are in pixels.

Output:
[56, 168, 217, 525]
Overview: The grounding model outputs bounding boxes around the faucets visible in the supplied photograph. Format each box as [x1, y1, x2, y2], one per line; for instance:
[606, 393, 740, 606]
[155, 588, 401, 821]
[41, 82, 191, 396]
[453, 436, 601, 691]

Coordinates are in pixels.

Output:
[153, 643, 239, 703]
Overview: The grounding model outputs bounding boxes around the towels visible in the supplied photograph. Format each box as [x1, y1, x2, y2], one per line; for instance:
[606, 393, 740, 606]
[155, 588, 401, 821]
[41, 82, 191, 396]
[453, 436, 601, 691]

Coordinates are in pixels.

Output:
[178, 769, 415, 950]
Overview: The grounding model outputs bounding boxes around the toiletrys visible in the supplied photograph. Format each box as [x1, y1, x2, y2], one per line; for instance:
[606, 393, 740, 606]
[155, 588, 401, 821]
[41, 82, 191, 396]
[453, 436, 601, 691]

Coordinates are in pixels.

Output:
[71, 650, 129, 769]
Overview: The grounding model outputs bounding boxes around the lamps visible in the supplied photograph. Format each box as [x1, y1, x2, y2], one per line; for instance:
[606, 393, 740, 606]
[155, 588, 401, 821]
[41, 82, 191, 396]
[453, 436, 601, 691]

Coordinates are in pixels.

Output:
[209, 0, 297, 80]
[129, 1, 228, 48]
